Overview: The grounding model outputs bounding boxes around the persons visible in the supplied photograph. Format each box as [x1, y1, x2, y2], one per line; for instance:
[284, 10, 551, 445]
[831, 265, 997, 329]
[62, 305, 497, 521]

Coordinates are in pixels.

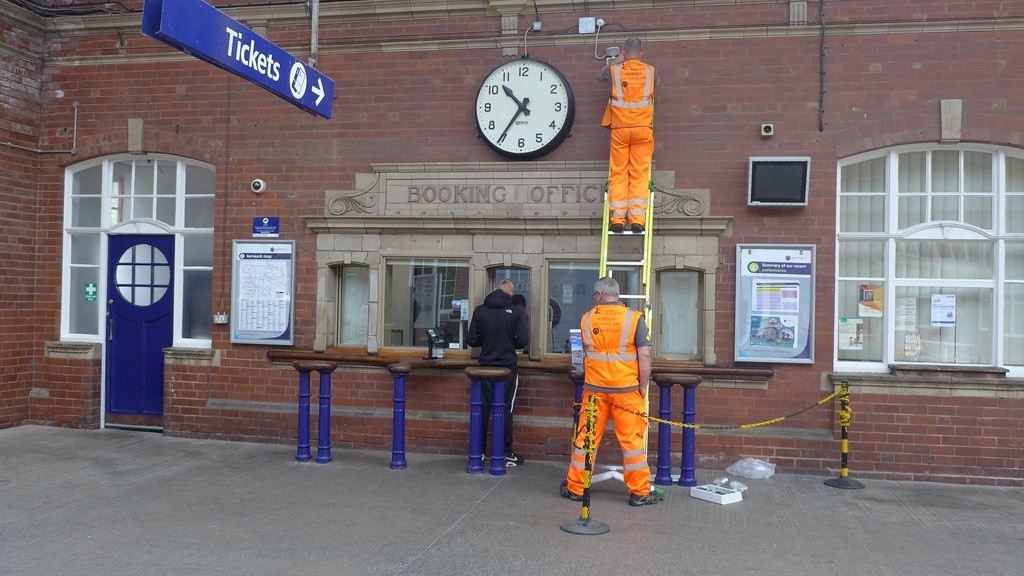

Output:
[466, 279, 529, 468]
[560, 276, 659, 506]
[596, 37, 660, 233]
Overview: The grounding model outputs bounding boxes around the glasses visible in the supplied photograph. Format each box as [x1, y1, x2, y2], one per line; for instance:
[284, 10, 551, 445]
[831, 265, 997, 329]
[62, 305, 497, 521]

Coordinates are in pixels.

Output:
[592, 291, 603, 299]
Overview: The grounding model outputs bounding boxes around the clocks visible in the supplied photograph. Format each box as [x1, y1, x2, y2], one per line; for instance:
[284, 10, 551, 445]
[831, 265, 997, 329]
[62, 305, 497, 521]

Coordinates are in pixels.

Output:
[474, 57, 576, 161]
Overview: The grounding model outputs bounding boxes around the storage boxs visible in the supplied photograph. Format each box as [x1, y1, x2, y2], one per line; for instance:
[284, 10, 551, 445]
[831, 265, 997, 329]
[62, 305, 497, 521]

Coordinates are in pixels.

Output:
[690, 484, 744, 505]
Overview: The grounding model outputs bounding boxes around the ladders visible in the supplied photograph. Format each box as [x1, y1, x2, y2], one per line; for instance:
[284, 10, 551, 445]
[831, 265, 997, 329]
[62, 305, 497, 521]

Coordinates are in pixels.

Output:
[589, 161, 656, 483]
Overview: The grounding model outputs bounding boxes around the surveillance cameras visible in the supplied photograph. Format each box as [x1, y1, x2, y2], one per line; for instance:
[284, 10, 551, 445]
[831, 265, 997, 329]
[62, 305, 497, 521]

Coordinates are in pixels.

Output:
[251, 179, 266, 193]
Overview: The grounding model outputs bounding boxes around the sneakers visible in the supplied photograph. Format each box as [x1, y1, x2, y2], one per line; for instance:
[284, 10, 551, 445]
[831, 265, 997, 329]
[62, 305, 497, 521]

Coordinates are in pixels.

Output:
[481, 454, 490, 465]
[629, 490, 660, 507]
[503, 451, 524, 468]
[560, 480, 584, 501]
